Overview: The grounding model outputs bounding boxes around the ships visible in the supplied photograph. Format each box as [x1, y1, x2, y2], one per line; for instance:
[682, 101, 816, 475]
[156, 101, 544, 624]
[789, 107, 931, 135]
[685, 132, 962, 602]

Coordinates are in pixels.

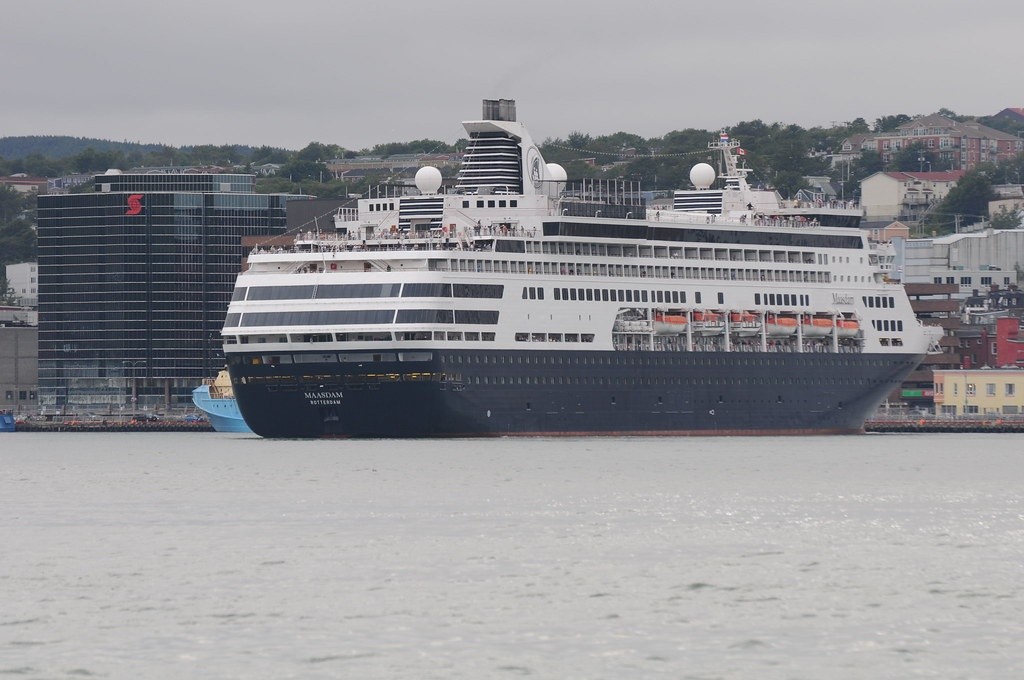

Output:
[220, 100, 943, 439]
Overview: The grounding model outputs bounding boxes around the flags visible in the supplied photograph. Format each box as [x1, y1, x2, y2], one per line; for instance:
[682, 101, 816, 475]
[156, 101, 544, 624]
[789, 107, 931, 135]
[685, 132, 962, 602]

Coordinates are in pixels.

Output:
[737, 148, 747, 156]
[721, 135, 729, 142]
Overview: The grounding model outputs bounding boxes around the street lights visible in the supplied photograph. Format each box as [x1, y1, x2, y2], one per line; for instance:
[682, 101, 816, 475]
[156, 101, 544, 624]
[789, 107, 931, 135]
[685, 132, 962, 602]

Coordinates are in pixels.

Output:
[123, 360, 146, 416]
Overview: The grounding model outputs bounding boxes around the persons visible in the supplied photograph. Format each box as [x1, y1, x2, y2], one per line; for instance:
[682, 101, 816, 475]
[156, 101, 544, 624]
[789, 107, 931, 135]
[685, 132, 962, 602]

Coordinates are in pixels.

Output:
[484, 224, 536, 237]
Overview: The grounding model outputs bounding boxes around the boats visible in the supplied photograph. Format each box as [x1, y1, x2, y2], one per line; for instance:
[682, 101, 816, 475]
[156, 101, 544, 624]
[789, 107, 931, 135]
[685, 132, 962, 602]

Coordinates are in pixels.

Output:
[654, 308, 860, 338]
[0, 409, 15, 432]
[192, 370, 255, 435]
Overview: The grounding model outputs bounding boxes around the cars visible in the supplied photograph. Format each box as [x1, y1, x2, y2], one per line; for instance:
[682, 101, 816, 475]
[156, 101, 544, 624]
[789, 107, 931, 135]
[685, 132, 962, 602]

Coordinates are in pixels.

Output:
[76, 412, 101, 421]
[133, 413, 156, 422]
[184, 414, 203, 422]
